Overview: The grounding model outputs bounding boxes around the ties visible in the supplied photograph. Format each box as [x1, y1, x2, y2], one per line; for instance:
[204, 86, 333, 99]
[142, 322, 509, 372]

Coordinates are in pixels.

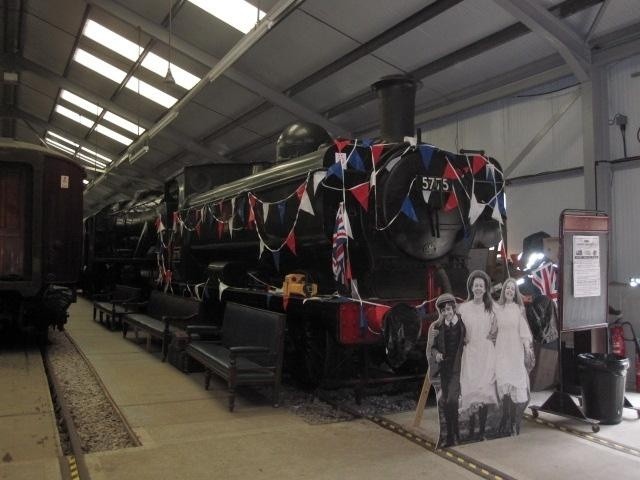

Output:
[450, 322, 453, 327]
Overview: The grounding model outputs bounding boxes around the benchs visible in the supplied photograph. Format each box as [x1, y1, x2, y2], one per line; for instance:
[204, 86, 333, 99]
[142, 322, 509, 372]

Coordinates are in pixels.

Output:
[93, 284, 141, 330]
[184, 299, 287, 410]
[123, 290, 204, 359]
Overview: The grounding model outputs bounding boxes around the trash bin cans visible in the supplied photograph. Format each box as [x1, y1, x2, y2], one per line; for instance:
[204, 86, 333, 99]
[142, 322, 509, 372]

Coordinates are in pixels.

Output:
[576, 352, 630, 425]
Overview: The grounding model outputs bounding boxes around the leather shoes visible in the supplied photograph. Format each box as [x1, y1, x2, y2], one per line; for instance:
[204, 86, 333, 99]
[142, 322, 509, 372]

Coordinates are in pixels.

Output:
[441, 435, 460, 448]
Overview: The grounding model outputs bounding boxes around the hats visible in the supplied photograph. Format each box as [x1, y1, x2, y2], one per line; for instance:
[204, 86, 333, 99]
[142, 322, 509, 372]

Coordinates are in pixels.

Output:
[436, 294, 455, 306]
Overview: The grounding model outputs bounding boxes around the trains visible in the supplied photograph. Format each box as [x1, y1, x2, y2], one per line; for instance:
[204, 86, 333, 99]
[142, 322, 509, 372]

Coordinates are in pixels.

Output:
[80, 73, 509, 407]
[0, 137, 89, 353]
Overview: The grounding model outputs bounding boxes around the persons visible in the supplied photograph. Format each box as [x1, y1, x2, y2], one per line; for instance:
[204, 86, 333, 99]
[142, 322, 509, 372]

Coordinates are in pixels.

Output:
[463, 278, 535, 435]
[429, 268, 526, 443]
[429, 292, 494, 448]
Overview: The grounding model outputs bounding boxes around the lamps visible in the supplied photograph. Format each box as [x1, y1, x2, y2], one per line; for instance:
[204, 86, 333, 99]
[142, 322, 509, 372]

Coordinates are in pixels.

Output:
[163, 0, 175, 84]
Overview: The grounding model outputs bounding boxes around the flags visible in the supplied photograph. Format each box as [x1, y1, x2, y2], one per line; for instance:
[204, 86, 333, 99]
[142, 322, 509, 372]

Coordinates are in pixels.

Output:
[529, 263, 560, 316]
[330, 203, 349, 294]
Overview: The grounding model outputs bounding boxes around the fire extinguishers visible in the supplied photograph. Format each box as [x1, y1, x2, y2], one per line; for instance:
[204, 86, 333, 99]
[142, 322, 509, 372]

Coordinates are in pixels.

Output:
[610, 318, 625, 356]
[636, 340, 640, 393]
